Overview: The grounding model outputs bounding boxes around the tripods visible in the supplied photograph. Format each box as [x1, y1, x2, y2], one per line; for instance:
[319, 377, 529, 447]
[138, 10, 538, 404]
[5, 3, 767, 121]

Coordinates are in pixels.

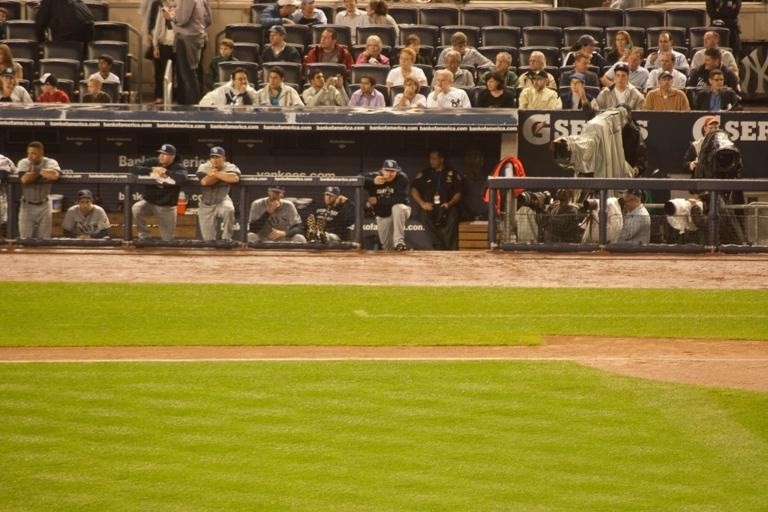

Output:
[576, 209, 599, 242]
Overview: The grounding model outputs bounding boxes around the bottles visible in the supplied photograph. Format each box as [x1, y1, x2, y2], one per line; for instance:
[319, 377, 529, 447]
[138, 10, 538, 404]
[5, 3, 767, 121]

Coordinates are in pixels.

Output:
[177, 192, 187, 216]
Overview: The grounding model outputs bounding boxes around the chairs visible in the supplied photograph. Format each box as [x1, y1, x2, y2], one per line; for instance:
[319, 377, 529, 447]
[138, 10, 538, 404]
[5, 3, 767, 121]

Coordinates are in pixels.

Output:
[211, 1, 737, 108]
[1, 0, 134, 103]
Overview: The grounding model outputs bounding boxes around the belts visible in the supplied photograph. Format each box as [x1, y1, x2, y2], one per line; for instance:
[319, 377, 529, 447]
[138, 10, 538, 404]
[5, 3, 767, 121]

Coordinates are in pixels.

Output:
[22, 199, 49, 205]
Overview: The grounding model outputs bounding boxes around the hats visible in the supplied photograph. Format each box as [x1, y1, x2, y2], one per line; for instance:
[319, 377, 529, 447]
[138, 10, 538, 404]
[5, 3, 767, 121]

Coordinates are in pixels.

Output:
[578, 35, 599, 45]
[156, 144, 177, 155]
[1, 69, 16, 76]
[32, 73, 57, 85]
[569, 72, 585, 83]
[704, 115, 718, 127]
[268, 25, 286, 33]
[209, 147, 225, 158]
[658, 71, 673, 79]
[77, 190, 94, 200]
[278, 0, 301, 6]
[382, 160, 402, 172]
[324, 186, 340, 198]
[268, 185, 285, 192]
[534, 71, 547, 78]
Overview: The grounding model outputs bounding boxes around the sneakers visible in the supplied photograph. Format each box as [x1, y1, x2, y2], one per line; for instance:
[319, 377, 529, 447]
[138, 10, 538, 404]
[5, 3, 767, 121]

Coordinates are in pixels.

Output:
[306, 212, 327, 243]
[395, 244, 407, 250]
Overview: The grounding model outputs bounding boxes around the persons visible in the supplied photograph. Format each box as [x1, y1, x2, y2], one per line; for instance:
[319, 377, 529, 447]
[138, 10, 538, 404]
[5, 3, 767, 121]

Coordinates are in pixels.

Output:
[90, 54, 120, 84]
[1, 44, 34, 102]
[360, 159, 413, 250]
[201, 0, 430, 108]
[130, 143, 188, 241]
[196, 146, 240, 241]
[1, 8, 7, 40]
[305, 186, 356, 243]
[34, 1, 96, 43]
[705, 1, 742, 53]
[17, 141, 63, 238]
[247, 184, 307, 244]
[64, 189, 111, 239]
[1, 153, 18, 246]
[409, 149, 465, 250]
[538, 103, 720, 246]
[425, 30, 743, 110]
[82, 78, 112, 103]
[37, 72, 72, 103]
[141, 0, 212, 104]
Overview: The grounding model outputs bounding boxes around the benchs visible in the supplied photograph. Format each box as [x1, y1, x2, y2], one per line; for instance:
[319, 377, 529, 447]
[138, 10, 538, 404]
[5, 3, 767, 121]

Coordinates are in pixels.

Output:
[53, 210, 198, 239]
[458, 219, 582, 251]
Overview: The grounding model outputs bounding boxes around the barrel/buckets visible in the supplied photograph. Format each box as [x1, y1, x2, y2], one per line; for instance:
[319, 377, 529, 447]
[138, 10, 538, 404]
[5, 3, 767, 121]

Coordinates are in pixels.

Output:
[49, 193, 63, 213]
[177, 195, 188, 214]
